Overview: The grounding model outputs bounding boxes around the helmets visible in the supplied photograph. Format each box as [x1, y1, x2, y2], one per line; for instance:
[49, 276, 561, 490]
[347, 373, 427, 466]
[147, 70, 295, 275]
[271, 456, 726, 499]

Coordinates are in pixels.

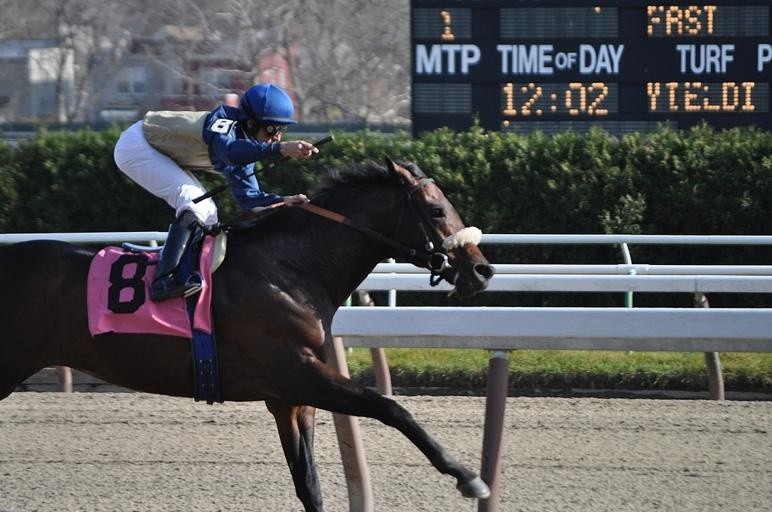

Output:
[238, 83, 298, 124]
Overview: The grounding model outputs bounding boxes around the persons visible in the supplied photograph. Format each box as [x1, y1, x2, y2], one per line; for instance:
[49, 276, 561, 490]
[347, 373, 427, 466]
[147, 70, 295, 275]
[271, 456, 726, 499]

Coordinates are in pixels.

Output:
[114, 82, 319, 303]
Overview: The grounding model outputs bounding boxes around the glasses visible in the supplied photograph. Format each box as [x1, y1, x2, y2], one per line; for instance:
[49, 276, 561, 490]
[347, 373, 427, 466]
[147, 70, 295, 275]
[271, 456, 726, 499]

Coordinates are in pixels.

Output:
[265, 124, 288, 136]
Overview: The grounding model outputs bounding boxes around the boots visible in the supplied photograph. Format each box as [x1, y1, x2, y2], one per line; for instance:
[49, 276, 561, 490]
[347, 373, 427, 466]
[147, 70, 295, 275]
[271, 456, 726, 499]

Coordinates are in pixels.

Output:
[150, 209, 203, 302]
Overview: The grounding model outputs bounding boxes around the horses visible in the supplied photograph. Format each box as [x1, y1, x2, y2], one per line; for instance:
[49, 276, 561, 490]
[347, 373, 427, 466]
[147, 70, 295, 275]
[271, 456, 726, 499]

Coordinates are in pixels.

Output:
[0, 152, 497, 511]
